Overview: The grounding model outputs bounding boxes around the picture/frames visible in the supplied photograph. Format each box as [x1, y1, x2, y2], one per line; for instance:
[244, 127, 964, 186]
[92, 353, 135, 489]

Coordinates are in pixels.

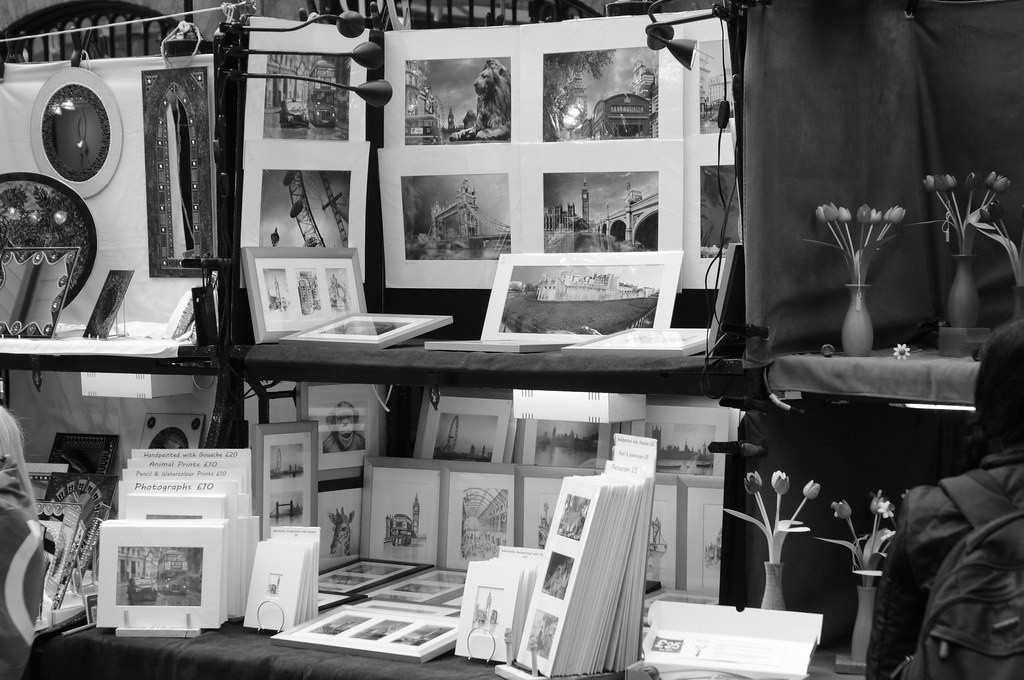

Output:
[0, 172, 723, 662]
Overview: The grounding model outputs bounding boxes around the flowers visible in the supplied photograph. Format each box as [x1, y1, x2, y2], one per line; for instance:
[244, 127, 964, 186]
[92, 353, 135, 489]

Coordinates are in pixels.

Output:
[903, 169, 1013, 257]
[814, 488, 911, 586]
[972, 200, 1024, 287]
[892, 343, 923, 360]
[722, 471, 820, 562]
[802, 202, 907, 282]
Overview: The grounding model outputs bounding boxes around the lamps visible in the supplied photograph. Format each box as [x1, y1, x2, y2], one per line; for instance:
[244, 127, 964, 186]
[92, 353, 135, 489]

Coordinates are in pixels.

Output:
[233, 73, 393, 108]
[231, 42, 383, 71]
[232, 10, 364, 38]
[644, 0, 732, 70]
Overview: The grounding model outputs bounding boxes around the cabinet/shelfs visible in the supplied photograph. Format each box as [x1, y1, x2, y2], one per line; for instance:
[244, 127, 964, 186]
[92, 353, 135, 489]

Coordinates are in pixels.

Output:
[1, 344, 873, 680]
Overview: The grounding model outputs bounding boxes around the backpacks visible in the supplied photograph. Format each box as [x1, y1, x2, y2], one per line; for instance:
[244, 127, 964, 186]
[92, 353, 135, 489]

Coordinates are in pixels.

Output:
[890, 466, 1024, 680]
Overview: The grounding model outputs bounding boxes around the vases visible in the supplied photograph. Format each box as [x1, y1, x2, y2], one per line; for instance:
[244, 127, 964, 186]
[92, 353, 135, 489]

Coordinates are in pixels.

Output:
[949, 253, 981, 327]
[1011, 285, 1024, 324]
[850, 587, 875, 661]
[840, 283, 874, 356]
[760, 562, 785, 610]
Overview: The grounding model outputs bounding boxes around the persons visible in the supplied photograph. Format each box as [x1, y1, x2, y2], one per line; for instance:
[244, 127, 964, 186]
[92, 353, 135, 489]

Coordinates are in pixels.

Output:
[864, 323, 1024, 680]
[0, 404, 44, 680]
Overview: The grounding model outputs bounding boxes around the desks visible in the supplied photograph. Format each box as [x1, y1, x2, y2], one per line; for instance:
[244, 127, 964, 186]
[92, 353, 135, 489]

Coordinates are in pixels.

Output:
[766, 353, 989, 478]
